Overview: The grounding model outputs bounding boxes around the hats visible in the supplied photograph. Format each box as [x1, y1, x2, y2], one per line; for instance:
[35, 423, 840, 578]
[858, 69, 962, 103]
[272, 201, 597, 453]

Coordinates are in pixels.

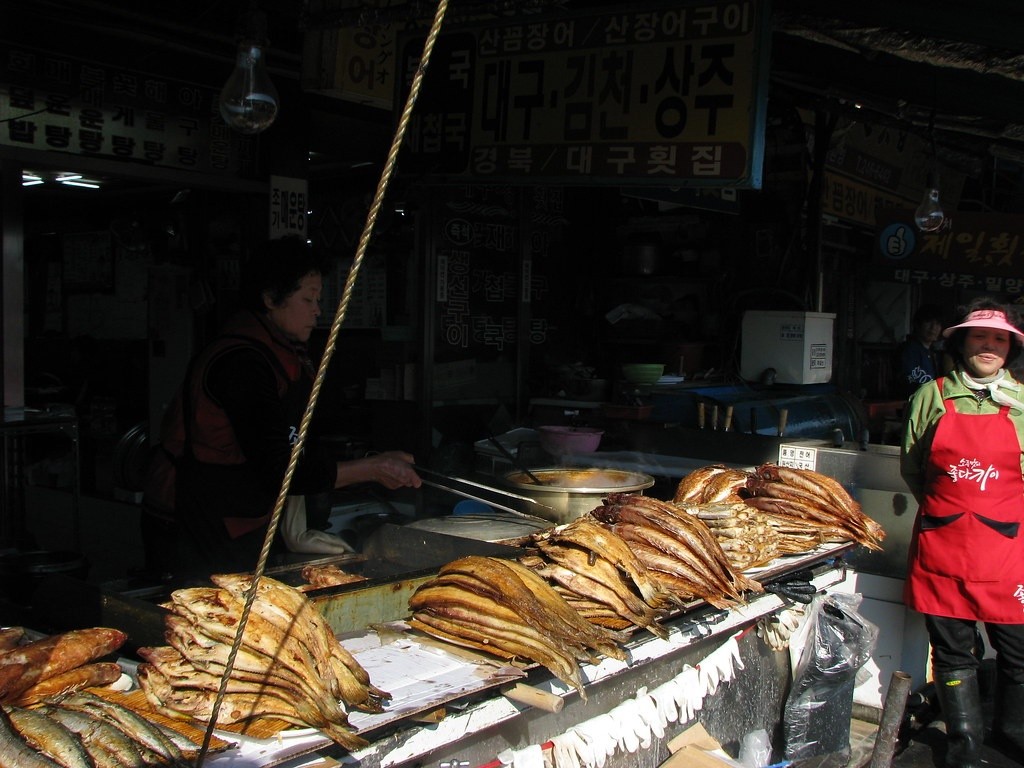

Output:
[942, 308, 1024, 348]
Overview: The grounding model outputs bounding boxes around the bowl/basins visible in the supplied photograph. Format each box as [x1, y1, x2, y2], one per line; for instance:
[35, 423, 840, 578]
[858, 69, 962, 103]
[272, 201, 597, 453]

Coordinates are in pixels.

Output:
[538, 424, 605, 456]
[500, 467, 656, 529]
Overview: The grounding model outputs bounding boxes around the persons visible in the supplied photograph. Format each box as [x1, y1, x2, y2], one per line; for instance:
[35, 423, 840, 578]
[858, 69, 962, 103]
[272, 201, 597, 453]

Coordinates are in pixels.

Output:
[891, 305, 969, 397]
[899, 296, 1024, 768]
[145, 250, 422, 575]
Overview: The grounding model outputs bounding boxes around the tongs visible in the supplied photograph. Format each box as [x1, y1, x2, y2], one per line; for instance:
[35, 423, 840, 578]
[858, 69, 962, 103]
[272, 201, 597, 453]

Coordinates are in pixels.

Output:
[363, 450, 558, 522]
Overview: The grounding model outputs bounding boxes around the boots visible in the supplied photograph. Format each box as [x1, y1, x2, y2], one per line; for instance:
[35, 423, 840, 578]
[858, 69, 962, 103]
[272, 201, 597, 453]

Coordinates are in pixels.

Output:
[930, 668, 985, 768]
[993, 683, 1024, 757]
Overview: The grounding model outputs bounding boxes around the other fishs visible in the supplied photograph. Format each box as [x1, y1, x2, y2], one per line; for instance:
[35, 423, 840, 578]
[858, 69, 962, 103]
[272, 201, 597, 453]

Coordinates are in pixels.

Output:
[0, 573, 395, 768]
[408, 459, 888, 706]
